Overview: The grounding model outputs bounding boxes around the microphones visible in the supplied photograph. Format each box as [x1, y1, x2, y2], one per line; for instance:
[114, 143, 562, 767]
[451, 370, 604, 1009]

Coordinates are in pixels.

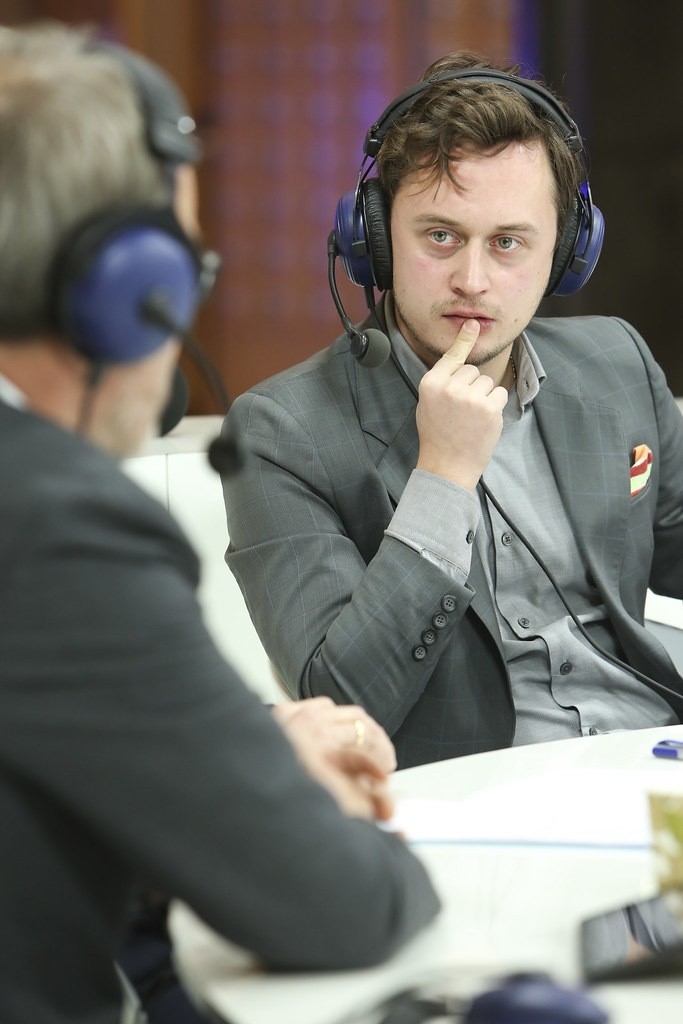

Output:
[141, 291, 247, 476]
[327, 229, 390, 368]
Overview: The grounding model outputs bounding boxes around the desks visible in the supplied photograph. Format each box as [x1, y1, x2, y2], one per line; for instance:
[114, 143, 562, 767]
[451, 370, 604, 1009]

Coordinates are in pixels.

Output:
[166, 723, 683, 1024]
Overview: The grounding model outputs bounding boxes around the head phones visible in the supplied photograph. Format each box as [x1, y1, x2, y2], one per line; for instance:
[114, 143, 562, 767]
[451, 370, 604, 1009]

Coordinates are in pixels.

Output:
[335, 68, 605, 297]
[49, 41, 200, 365]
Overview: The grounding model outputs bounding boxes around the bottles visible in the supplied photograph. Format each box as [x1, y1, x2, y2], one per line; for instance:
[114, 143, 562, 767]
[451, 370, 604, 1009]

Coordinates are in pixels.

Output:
[651, 737, 683, 891]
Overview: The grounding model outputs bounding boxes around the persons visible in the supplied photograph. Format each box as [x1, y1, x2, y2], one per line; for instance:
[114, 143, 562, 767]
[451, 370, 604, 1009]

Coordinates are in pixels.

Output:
[0, 27, 445, 1024]
[216, 53, 683, 773]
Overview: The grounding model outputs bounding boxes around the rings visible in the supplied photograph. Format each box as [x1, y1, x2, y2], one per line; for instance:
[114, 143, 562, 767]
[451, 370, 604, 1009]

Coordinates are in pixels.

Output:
[351, 719, 367, 749]
